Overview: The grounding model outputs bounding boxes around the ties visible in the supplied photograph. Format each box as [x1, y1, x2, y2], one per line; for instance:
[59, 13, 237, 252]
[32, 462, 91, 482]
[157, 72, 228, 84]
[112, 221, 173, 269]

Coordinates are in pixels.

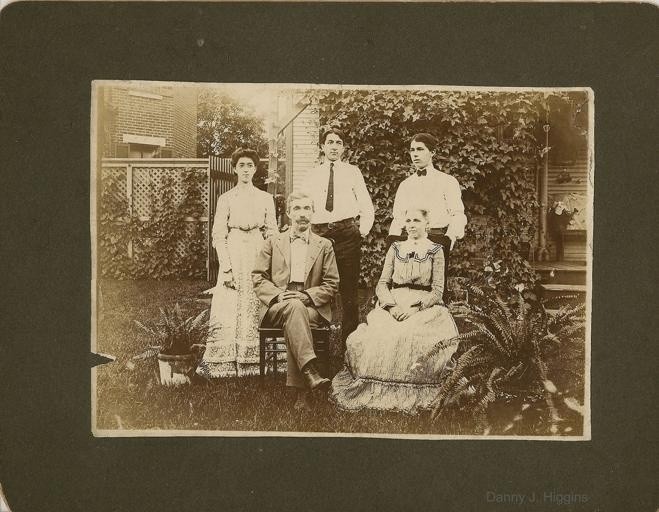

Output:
[325, 163, 334, 212]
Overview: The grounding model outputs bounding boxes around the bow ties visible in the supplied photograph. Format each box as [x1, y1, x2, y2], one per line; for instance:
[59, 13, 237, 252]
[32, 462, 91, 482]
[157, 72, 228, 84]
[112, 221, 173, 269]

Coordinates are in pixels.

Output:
[417, 169, 426, 176]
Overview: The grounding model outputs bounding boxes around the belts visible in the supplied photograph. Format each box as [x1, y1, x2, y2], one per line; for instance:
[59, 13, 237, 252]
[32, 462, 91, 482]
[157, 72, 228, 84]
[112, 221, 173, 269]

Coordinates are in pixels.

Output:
[318, 218, 353, 232]
[429, 229, 445, 237]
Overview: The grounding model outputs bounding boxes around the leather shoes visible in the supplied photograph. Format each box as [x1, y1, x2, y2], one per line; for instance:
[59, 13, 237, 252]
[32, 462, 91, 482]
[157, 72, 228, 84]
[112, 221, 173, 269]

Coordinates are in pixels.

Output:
[303, 367, 331, 391]
[294, 392, 311, 411]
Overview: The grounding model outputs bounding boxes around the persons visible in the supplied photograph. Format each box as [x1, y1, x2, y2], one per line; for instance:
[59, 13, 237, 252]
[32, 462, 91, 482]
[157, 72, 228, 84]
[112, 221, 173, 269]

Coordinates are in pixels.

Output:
[252, 187, 339, 402]
[298, 127, 374, 360]
[192, 146, 293, 377]
[388, 131, 469, 300]
[328, 205, 475, 412]
